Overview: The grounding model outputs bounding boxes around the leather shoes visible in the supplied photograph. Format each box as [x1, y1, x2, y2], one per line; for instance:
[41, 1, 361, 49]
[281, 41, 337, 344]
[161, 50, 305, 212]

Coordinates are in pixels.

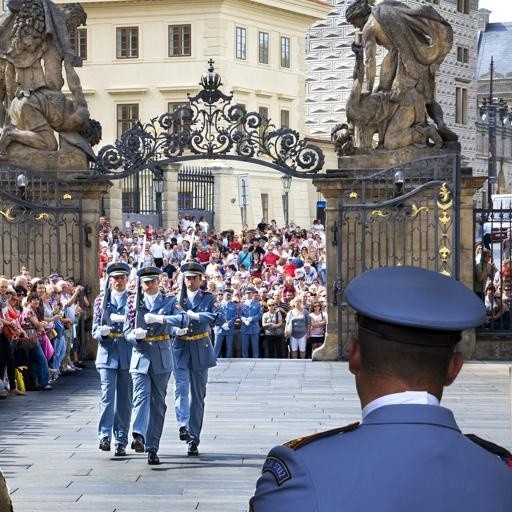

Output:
[98, 427, 198, 465]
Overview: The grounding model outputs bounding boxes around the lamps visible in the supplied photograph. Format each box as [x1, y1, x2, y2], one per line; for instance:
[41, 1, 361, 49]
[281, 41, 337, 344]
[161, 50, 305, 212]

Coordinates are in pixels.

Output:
[16, 173, 30, 215]
[390, 169, 409, 208]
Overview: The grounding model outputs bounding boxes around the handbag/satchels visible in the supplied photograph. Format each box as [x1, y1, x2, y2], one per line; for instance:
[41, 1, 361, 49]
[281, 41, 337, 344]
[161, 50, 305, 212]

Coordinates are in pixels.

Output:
[276, 327, 283, 336]
[284, 321, 293, 340]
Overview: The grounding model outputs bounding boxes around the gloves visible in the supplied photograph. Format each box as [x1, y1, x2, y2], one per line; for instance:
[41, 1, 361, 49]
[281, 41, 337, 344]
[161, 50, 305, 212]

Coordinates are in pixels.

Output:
[187, 310, 199, 320]
[242, 317, 253, 325]
[129, 328, 147, 339]
[143, 312, 165, 325]
[110, 312, 127, 323]
[98, 325, 110, 337]
[173, 326, 188, 336]
[221, 322, 230, 331]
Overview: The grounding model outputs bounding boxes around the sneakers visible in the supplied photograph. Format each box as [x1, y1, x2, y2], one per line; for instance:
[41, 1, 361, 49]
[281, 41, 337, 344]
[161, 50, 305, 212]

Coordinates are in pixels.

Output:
[43, 360, 87, 389]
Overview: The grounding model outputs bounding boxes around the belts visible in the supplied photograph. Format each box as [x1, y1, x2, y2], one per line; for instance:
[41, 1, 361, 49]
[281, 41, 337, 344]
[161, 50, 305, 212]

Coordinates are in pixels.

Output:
[143, 334, 171, 342]
[176, 332, 209, 341]
[109, 332, 123, 337]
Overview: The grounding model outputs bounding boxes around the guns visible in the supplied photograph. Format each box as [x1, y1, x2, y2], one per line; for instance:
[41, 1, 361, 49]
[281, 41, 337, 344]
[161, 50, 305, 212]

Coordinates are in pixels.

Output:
[101, 243, 117, 339]
[134, 234, 146, 343]
[179, 230, 195, 310]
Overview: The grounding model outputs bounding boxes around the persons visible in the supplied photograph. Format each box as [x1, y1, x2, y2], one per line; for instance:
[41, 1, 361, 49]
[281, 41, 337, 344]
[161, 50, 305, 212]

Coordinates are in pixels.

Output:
[91, 262, 134, 456]
[346, 0, 459, 141]
[0, 0, 88, 125]
[0, 48, 102, 154]
[248, 266, 512, 512]
[98, 212, 327, 359]
[0, 266, 90, 397]
[475, 227, 510, 332]
[168, 262, 225, 457]
[331, 41, 443, 157]
[122, 265, 189, 464]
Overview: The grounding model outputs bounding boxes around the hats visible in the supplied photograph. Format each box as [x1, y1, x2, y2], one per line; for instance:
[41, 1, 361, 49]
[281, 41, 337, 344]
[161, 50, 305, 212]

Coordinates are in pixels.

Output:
[343, 265, 486, 347]
[224, 287, 234, 295]
[181, 262, 205, 278]
[107, 263, 131, 276]
[243, 286, 255, 294]
[137, 266, 161, 282]
